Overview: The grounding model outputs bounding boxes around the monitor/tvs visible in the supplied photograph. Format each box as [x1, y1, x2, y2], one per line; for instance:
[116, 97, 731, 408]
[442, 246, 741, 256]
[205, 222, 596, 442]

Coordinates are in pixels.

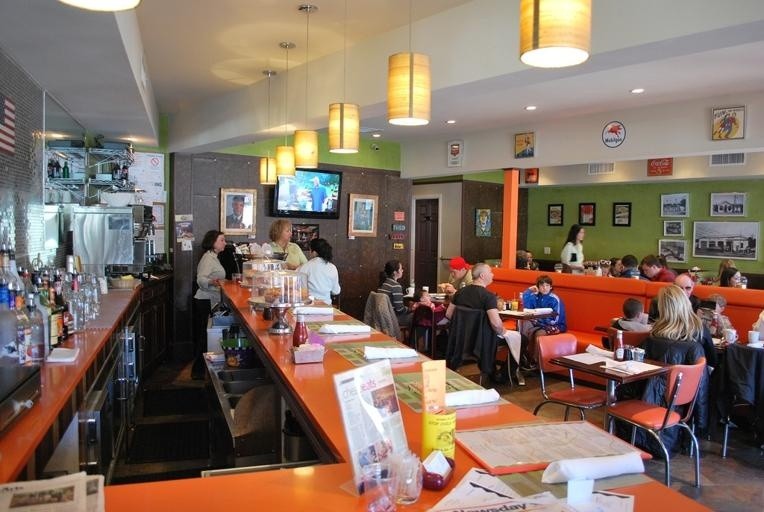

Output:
[273, 169, 342, 220]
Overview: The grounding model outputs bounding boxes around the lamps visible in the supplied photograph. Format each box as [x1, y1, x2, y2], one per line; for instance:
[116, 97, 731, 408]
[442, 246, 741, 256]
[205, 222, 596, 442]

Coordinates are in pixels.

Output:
[259, 1, 431, 185]
[518, 0, 594, 70]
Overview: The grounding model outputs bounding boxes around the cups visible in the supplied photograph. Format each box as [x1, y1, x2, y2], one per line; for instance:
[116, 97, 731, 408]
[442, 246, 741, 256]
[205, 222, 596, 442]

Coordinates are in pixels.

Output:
[723, 328, 738, 344]
[748, 331, 759, 343]
[423, 286, 429, 293]
[51, 190, 72, 203]
[736, 276, 747, 288]
[624, 344, 645, 361]
[99, 278, 108, 295]
[389, 458, 423, 504]
[406, 287, 415, 297]
[232, 273, 241, 283]
[362, 463, 397, 512]
[496, 296, 518, 311]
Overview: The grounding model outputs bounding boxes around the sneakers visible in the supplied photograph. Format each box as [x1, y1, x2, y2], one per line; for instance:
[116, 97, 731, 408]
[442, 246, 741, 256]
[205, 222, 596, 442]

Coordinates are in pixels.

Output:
[521, 361, 536, 370]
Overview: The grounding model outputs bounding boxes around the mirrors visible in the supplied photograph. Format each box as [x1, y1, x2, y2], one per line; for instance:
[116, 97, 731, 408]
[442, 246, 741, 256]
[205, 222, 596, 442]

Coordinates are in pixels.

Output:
[43, 90, 87, 207]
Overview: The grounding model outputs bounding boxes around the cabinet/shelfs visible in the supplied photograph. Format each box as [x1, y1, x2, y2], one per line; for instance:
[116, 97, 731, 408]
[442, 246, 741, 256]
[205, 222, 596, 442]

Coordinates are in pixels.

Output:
[46, 147, 86, 207]
[88, 146, 135, 199]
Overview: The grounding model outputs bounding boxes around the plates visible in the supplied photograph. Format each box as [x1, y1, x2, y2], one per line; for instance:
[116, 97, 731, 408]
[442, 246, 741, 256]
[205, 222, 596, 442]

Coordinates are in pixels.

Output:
[436, 297, 446, 300]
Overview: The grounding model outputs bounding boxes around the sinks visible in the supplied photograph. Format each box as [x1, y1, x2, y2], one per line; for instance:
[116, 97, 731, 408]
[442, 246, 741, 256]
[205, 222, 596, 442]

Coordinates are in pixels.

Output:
[216, 382, 273, 395]
[214, 369, 267, 382]
[218, 395, 242, 423]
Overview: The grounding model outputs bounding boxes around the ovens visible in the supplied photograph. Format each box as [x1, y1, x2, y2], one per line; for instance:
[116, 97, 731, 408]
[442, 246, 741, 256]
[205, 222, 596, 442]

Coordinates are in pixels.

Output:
[123, 302, 145, 427]
[78, 353, 125, 485]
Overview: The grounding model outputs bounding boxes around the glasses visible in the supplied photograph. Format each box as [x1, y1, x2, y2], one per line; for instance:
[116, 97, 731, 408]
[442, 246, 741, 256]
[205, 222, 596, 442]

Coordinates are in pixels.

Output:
[679, 287, 691, 291]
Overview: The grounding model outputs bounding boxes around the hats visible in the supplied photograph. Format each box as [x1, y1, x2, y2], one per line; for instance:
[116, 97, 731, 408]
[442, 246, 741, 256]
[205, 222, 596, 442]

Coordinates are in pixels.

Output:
[449, 257, 472, 271]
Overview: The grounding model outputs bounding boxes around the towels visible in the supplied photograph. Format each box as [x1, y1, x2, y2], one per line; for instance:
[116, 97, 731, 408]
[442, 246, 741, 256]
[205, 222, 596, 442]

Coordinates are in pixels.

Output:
[318, 324, 371, 335]
[45, 347, 80, 363]
[292, 306, 334, 315]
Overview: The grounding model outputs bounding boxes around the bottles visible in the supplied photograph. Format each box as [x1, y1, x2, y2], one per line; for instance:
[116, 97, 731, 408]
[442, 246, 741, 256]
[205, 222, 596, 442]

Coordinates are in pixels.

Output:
[293, 312, 308, 346]
[233, 242, 273, 255]
[518, 292, 524, 311]
[112, 161, 128, 181]
[614, 330, 624, 360]
[47, 155, 69, 178]
[0, 242, 100, 365]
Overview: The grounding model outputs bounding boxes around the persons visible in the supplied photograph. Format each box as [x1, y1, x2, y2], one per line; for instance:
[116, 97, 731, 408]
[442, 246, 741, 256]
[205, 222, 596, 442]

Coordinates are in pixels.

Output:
[296, 238, 341, 306]
[477, 211, 490, 235]
[521, 276, 567, 371]
[193, 230, 227, 366]
[269, 219, 308, 270]
[445, 262, 522, 384]
[302, 176, 328, 213]
[226, 195, 246, 228]
[713, 112, 738, 138]
[600, 274, 763, 429]
[443, 256, 475, 301]
[376, 259, 413, 327]
[517, 225, 749, 289]
[411, 289, 447, 328]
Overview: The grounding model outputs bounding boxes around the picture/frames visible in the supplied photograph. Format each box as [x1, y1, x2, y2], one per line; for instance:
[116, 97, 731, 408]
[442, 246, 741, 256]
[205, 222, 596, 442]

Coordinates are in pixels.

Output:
[219, 187, 257, 236]
[548, 202, 632, 227]
[525, 168, 539, 184]
[514, 132, 535, 159]
[347, 193, 379, 238]
[711, 104, 746, 141]
[291, 223, 319, 251]
[657, 192, 760, 265]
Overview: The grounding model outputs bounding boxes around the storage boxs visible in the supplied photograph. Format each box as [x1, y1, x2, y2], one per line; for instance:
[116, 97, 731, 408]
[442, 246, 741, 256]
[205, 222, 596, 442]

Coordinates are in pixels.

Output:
[206, 313, 241, 352]
